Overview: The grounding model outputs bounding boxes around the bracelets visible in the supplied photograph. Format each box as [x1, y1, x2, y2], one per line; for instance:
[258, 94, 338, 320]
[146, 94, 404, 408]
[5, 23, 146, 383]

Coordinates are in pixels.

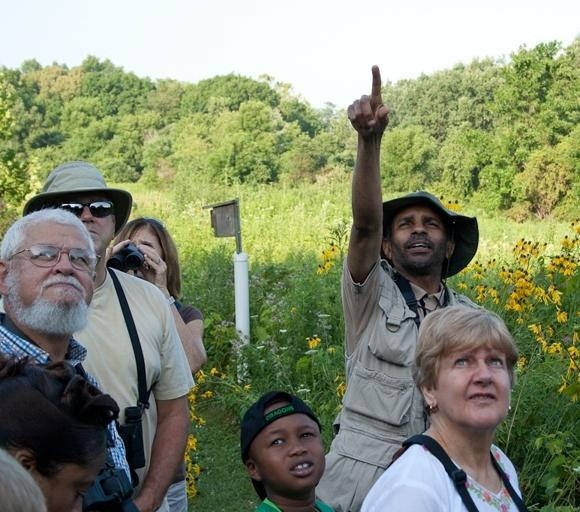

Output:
[167, 296, 176, 306]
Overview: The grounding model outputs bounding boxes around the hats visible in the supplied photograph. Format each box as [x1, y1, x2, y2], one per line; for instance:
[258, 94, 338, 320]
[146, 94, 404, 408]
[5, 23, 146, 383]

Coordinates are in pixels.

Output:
[383, 190, 479, 279]
[240, 391, 322, 502]
[23, 160, 132, 238]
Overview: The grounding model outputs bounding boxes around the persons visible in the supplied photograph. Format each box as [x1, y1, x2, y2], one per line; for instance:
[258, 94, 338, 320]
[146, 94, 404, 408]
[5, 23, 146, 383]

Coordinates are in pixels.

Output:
[98, 213, 207, 511]
[1, 351, 120, 512]
[25, 163, 194, 512]
[0, 206, 136, 512]
[356, 304, 532, 510]
[0, 447, 49, 512]
[316, 63, 482, 510]
[238, 388, 341, 511]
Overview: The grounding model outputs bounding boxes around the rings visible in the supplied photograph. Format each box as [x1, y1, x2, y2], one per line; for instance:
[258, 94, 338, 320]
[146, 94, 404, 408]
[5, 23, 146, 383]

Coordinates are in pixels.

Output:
[160, 258, 161, 264]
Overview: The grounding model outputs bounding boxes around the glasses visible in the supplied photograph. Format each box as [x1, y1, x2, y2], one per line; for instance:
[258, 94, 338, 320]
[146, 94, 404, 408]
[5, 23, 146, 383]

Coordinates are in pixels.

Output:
[7, 244, 102, 272]
[56, 197, 116, 218]
